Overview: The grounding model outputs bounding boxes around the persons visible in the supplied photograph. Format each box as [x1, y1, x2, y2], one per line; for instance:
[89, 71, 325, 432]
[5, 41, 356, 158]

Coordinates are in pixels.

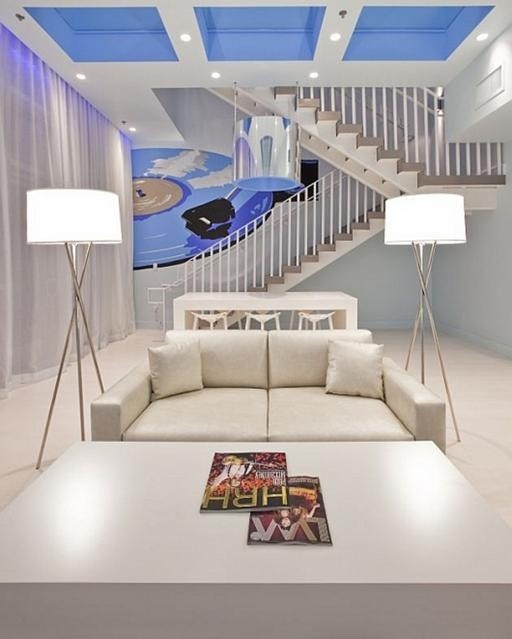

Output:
[210, 453, 256, 489]
[274, 482, 320, 530]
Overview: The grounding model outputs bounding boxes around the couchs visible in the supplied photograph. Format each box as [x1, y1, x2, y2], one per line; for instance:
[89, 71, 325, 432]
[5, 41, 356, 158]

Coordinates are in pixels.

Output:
[90, 329, 446, 456]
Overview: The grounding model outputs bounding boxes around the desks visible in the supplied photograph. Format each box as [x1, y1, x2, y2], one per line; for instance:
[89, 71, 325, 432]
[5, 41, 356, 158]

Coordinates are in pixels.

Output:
[173, 292, 358, 330]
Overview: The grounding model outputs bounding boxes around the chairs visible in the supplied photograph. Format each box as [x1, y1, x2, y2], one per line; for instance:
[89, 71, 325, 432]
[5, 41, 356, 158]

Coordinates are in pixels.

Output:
[189, 310, 336, 330]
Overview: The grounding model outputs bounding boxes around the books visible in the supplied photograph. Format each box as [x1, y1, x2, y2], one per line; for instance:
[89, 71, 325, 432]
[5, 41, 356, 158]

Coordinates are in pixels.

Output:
[200, 452, 288, 511]
[246, 476, 332, 545]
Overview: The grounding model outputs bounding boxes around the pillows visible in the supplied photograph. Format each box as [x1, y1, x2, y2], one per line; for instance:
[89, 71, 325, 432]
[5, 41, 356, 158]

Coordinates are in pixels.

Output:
[268, 329, 373, 389]
[325, 339, 385, 403]
[148, 338, 203, 401]
[166, 329, 268, 390]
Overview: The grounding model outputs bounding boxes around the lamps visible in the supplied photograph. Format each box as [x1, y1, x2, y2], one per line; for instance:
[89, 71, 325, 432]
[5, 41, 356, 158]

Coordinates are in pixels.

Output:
[384, 194, 466, 442]
[229, 81, 301, 192]
[26, 189, 123, 469]
